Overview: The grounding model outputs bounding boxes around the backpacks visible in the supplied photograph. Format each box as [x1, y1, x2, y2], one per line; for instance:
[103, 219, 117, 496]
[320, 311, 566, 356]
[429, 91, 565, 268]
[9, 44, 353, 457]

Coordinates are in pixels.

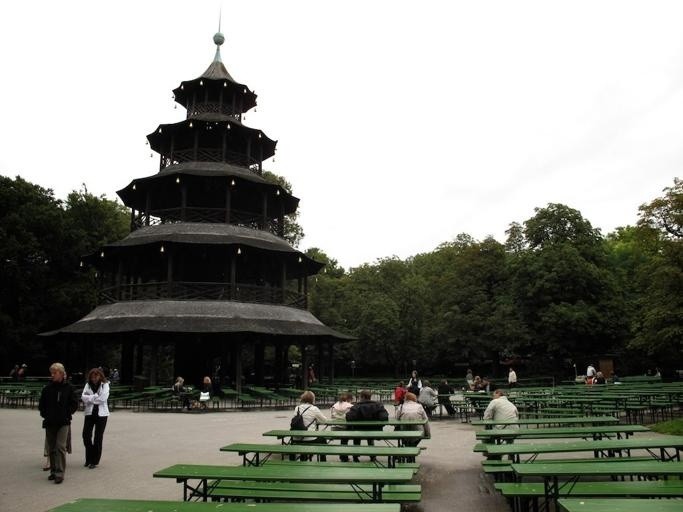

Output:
[288, 404, 319, 431]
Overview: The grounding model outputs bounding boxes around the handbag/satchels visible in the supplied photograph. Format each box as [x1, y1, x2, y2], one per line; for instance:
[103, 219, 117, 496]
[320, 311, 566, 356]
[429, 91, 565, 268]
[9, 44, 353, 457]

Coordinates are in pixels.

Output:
[393, 424, 402, 432]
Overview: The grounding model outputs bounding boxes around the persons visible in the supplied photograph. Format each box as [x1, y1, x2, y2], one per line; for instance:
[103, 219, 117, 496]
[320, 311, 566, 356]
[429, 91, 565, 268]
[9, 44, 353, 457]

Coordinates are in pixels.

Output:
[507, 367, 518, 389]
[199, 374, 215, 411]
[466, 368, 496, 416]
[418, 380, 437, 424]
[42, 370, 73, 472]
[331, 393, 356, 463]
[394, 381, 409, 407]
[38, 363, 79, 485]
[346, 391, 389, 463]
[9, 364, 20, 379]
[172, 376, 195, 411]
[407, 370, 424, 397]
[584, 363, 620, 384]
[18, 364, 29, 382]
[438, 379, 457, 417]
[483, 388, 521, 461]
[394, 392, 432, 464]
[291, 391, 328, 461]
[97, 366, 121, 381]
[81, 368, 111, 469]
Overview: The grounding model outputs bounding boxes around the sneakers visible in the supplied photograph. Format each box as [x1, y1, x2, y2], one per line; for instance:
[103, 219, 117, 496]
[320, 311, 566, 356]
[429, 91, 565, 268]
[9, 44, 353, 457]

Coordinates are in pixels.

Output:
[83, 461, 97, 469]
[46, 473, 55, 480]
[54, 476, 64, 484]
[41, 465, 50, 471]
